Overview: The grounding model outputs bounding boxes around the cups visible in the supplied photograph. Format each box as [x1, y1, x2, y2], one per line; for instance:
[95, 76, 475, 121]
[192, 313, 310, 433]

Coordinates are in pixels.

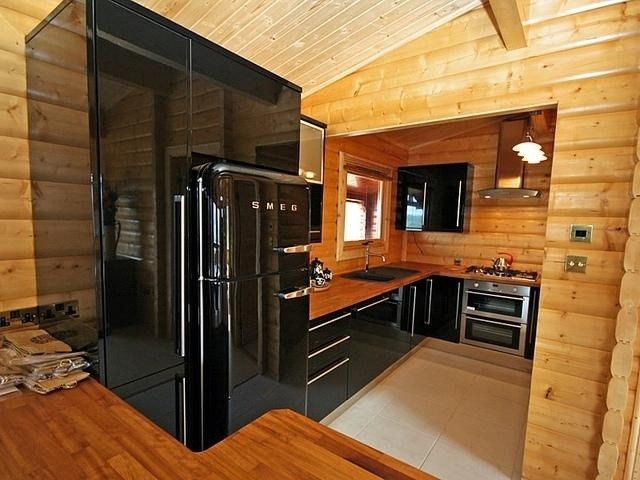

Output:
[454, 255, 463, 266]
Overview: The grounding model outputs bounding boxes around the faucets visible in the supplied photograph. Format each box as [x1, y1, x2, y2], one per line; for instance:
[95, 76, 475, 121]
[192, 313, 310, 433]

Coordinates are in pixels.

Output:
[362, 240, 386, 273]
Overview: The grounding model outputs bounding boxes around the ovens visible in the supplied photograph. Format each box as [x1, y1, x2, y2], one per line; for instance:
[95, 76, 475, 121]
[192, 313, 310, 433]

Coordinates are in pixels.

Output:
[460, 289, 537, 360]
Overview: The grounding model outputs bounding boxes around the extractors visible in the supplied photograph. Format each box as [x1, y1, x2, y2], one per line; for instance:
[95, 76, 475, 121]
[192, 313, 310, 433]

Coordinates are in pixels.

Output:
[474, 119, 543, 202]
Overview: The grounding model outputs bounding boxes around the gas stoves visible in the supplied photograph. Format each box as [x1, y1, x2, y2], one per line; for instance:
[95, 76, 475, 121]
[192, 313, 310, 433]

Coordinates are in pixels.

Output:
[466, 267, 538, 297]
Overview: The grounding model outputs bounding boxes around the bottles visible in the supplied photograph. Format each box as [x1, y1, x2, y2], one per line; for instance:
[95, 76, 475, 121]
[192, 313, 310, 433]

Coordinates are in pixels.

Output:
[309, 257, 333, 287]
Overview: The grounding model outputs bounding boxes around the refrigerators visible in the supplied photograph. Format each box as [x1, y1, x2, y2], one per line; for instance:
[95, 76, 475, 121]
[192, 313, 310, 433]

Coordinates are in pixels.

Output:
[193, 161, 310, 452]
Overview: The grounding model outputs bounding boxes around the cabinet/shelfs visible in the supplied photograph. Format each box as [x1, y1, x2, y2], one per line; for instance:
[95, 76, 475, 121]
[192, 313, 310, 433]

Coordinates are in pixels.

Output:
[24, 0, 193, 452]
[395, 163, 475, 233]
[190, 23, 302, 175]
[307, 275, 464, 421]
[524, 287, 541, 360]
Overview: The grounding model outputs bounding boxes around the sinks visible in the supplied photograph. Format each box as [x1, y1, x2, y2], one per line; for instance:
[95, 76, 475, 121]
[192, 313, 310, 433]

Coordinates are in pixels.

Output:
[340, 266, 419, 283]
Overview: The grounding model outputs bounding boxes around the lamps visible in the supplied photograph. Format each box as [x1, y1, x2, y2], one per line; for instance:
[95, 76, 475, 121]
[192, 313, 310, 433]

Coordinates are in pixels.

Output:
[512, 115, 548, 164]
